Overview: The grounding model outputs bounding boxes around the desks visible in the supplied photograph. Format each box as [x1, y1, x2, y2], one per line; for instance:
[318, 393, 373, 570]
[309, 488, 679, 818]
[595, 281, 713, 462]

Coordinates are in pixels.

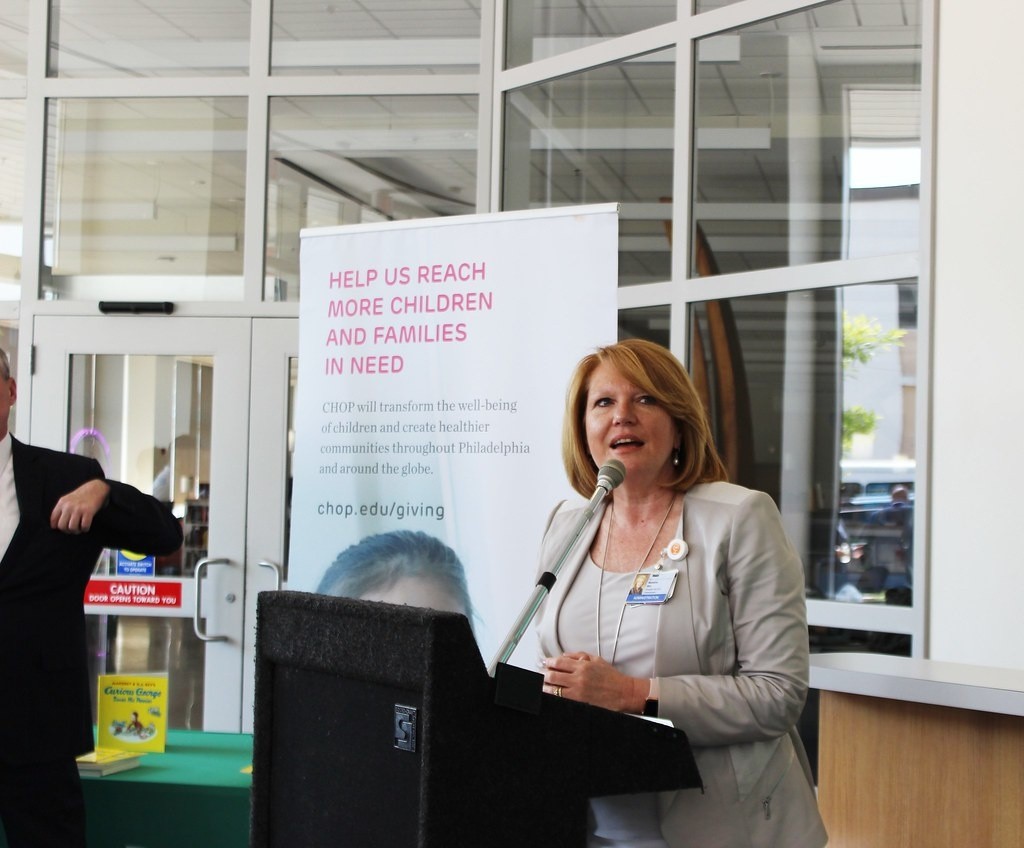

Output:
[76, 726, 255, 848]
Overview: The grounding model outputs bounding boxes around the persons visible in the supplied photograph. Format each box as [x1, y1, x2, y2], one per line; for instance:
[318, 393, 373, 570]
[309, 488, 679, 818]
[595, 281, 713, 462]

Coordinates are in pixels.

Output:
[314, 529, 477, 645]
[0, 348, 182, 848]
[534, 338, 828, 848]
[859, 487, 913, 588]
[153, 434, 197, 502]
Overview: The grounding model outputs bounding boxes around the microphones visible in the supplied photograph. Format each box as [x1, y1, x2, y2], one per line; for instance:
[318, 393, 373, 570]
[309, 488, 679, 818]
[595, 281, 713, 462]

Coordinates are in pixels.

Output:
[487, 458, 626, 677]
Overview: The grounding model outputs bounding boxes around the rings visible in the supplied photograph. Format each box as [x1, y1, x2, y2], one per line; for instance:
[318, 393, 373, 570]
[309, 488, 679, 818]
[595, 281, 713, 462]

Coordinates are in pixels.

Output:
[554, 687, 562, 697]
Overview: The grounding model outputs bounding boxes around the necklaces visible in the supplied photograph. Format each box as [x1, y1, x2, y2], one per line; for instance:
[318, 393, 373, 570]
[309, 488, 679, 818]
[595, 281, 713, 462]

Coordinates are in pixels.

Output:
[597, 495, 676, 667]
